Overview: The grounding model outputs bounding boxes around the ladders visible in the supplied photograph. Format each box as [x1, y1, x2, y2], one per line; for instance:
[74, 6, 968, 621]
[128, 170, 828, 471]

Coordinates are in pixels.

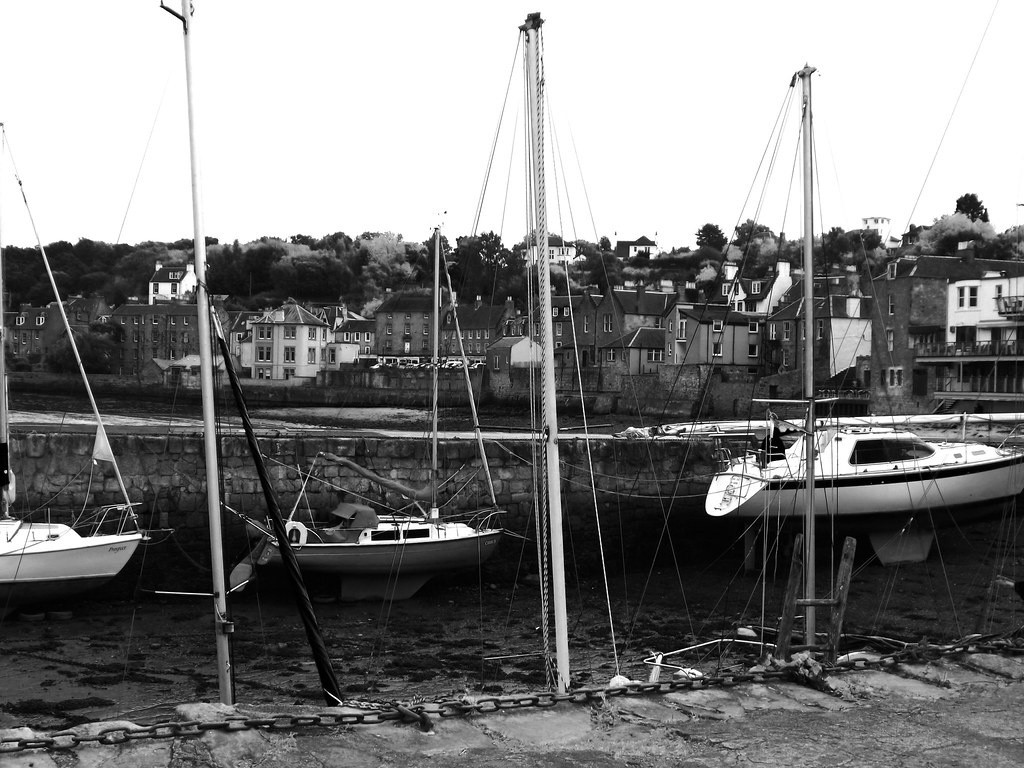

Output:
[775, 533, 859, 664]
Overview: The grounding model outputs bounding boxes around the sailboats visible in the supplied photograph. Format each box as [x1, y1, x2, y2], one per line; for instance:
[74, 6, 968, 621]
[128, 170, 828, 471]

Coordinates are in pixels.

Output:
[256, 224, 505, 602]
[0, 121, 145, 608]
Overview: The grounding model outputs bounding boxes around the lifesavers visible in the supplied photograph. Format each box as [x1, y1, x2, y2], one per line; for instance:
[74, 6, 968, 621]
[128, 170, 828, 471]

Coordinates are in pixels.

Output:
[283, 520, 308, 547]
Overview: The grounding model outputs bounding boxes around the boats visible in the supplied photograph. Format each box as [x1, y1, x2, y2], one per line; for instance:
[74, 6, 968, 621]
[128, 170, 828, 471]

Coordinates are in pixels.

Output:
[705, 423, 1024, 530]
